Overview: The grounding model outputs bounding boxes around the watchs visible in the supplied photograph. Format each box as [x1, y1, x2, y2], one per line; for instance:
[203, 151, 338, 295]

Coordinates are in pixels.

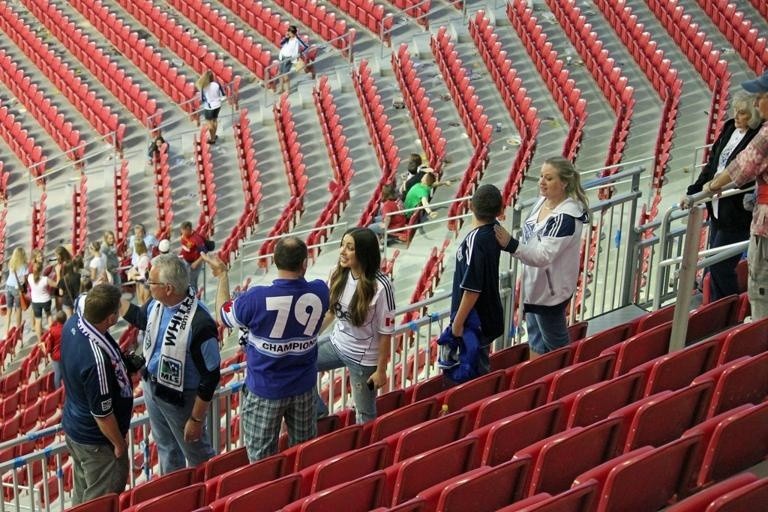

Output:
[189, 417, 203, 424]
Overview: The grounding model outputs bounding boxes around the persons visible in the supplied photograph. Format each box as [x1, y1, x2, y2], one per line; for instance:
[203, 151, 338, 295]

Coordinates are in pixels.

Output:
[62, 284, 136, 506]
[178, 221, 208, 269]
[317, 227, 397, 425]
[703, 69, 768, 323]
[275, 24, 308, 95]
[6, 246, 28, 338]
[59, 264, 78, 320]
[99, 231, 122, 285]
[51, 245, 72, 312]
[148, 137, 170, 164]
[27, 249, 44, 273]
[27, 264, 61, 341]
[117, 253, 221, 476]
[127, 222, 160, 260]
[131, 240, 151, 305]
[199, 235, 329, 464]
[89, 242, 109, 289]
[437, 185, 506, 378]
[194, 70, 225, 145]
[686, 88, 764, 304]
[368, 185, 407, 249]
[403, 172, 450, 218]
[495, 157, 586, 361]
[398, 153, 426, 197]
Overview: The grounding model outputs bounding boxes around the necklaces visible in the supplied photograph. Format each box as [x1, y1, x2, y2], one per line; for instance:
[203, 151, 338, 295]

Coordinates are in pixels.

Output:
[540, 201, 556, 211]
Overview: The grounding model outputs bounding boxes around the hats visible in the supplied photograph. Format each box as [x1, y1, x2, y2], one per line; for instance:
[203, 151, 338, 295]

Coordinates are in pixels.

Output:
[741, 71, 768, 94]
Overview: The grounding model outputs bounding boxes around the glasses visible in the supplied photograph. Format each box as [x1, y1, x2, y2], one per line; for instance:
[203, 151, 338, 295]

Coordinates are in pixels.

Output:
[146, 278, 164, 288]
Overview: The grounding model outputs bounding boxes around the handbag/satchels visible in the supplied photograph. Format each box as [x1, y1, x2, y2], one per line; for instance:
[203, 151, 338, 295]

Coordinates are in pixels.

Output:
[14, 266, 31, 311]
